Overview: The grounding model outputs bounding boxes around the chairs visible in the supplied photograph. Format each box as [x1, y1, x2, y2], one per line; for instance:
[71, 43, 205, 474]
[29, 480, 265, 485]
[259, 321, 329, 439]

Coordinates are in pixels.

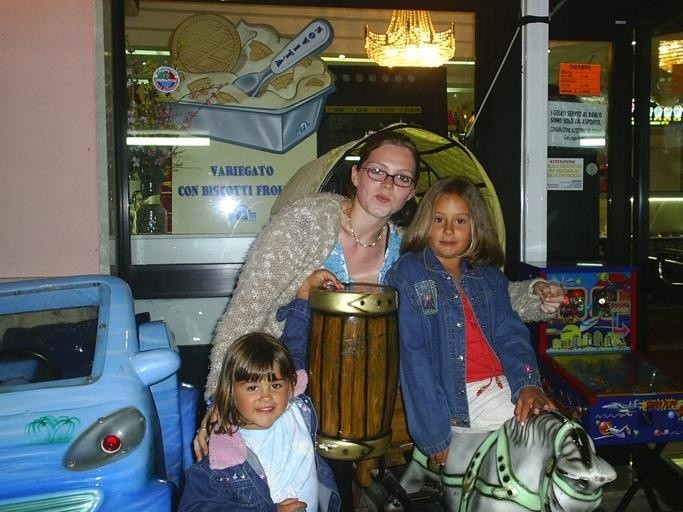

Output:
[0, 313, 149, 379]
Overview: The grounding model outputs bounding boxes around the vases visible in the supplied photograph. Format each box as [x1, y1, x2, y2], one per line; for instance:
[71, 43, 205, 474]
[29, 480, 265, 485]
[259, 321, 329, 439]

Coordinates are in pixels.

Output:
[132, 182, 169, 235]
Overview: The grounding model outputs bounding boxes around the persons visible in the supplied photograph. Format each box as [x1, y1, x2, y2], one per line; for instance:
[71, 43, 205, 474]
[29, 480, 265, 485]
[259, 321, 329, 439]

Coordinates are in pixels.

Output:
[177, 269, 345, 512]
[384, 175, 556, 467]
[192, 129, 565, 463]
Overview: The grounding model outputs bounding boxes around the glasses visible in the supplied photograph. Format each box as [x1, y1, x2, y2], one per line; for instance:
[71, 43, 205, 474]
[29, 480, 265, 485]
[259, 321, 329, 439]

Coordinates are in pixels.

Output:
[360, 166, 417, 188]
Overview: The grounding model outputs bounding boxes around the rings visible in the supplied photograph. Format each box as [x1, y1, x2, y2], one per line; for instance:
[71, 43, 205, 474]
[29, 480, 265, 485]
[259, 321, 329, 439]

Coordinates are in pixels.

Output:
[197, 426, 207, 433]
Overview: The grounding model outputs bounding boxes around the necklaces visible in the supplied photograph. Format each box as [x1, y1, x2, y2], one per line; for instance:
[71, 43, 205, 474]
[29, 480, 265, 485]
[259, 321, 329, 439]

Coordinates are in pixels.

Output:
[348, 203, 386, 248]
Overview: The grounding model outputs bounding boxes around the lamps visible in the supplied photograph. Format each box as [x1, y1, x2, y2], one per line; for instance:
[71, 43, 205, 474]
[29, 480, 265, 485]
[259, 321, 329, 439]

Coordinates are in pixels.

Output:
[365, 9, 456, 69]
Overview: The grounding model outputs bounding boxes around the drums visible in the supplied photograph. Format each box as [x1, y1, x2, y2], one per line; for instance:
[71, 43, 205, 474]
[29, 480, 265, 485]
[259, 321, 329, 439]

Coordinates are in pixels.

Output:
[305, 283, 400, 462]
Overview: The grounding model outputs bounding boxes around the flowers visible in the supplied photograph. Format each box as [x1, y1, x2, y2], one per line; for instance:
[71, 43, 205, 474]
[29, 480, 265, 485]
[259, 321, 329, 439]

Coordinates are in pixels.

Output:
[125, 31, 229, 184]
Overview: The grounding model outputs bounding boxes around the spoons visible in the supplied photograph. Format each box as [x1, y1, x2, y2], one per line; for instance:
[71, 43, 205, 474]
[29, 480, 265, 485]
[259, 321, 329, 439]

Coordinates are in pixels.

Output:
[231, 20, 331, 95]
